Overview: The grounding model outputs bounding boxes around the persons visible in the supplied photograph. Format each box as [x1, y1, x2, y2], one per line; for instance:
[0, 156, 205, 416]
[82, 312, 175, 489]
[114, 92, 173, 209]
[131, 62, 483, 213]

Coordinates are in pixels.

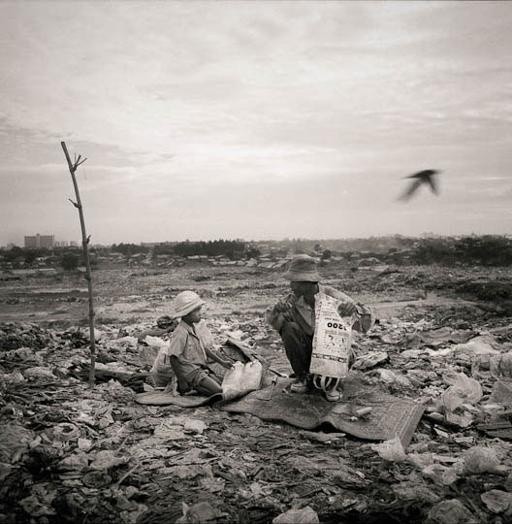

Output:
[261, 252, 374, 402]
[167, 289, 237, 397]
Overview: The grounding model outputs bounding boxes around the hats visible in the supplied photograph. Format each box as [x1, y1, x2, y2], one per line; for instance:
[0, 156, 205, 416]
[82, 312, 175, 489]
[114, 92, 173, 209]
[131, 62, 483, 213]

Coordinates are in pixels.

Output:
[170, 291, 205, 319]
[282, 253, 321, 282]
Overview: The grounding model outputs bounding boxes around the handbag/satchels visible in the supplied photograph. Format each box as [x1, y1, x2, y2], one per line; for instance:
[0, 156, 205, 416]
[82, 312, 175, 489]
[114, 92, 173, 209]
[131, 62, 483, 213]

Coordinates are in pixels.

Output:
[309, 291, 361, 379]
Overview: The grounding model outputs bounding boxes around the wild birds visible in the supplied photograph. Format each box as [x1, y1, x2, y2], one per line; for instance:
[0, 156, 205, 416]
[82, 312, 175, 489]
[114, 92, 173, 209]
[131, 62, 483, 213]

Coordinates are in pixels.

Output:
[401, 170, 441, 197]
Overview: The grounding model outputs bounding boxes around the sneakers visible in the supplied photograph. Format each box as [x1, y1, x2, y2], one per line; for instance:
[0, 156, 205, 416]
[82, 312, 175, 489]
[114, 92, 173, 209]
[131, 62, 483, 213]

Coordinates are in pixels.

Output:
[325, 386, 343, 402]
[291, 378, 309, 394]
